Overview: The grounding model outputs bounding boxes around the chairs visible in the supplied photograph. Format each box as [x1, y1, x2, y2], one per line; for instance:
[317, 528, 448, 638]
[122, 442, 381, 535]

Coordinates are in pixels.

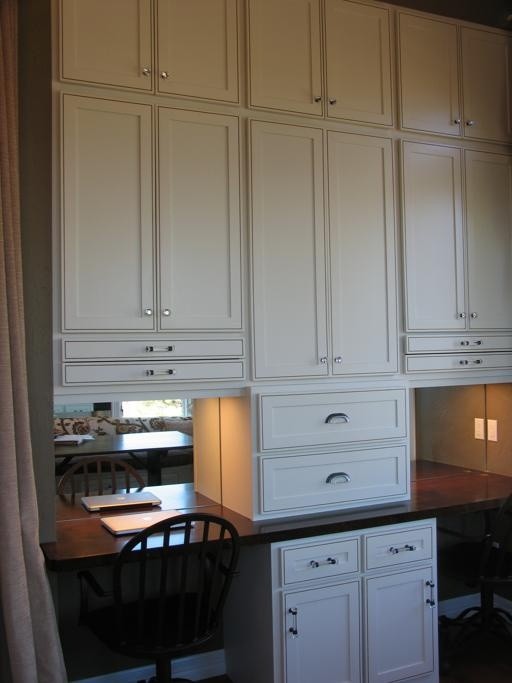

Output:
[72, 511, 242, 682]
[440, 492, 510, 678]
[56, 453, 146, 501]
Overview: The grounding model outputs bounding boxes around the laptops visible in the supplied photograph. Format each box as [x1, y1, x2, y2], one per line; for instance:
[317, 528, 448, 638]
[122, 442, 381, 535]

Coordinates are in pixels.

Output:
[80, 492, 160, 510]
[101, 510, 192, 538]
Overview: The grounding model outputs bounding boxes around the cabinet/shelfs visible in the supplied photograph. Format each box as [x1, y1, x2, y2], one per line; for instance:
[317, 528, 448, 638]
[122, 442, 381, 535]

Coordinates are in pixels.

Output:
[231, 515, 447, 683]
[49, 0, 512, 400]
[178, 390, 425, 518]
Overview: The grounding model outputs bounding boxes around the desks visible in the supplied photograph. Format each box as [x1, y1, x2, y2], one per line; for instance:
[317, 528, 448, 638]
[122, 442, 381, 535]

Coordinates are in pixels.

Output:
[41, 456, 512, 575]
[50, 426, 195, 485]
